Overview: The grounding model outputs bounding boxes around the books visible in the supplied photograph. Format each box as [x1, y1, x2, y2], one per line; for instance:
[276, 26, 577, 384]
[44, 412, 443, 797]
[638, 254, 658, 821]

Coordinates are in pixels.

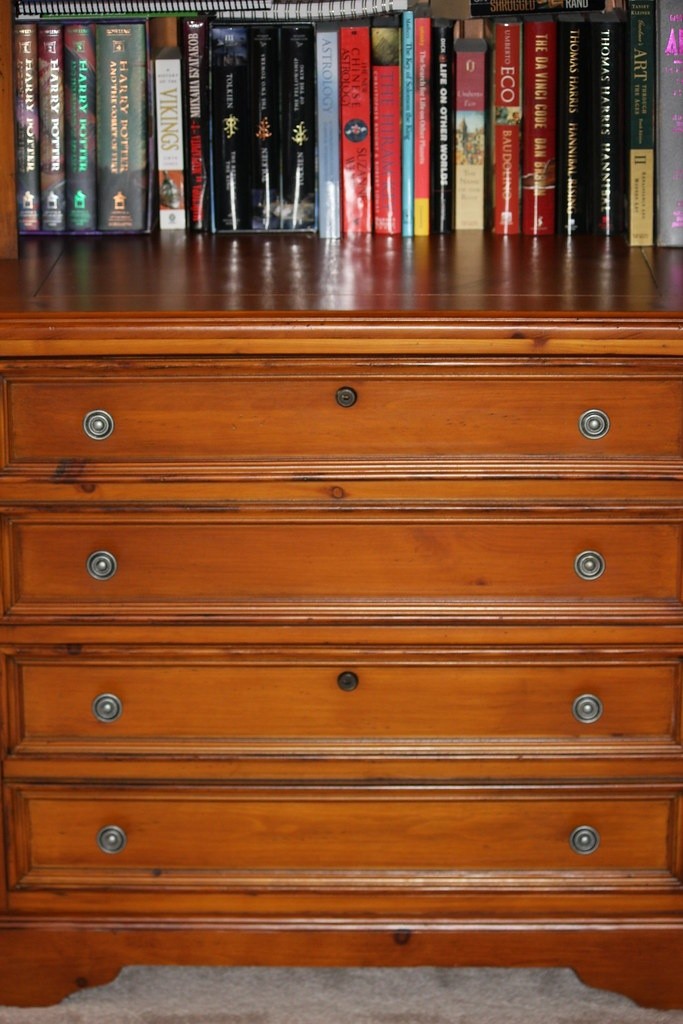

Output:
[11, 1, 683, 248]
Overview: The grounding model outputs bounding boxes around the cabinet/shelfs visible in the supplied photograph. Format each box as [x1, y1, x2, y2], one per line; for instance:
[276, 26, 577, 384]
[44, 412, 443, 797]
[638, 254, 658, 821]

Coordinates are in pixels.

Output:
[0, 0, 683, 1009]
